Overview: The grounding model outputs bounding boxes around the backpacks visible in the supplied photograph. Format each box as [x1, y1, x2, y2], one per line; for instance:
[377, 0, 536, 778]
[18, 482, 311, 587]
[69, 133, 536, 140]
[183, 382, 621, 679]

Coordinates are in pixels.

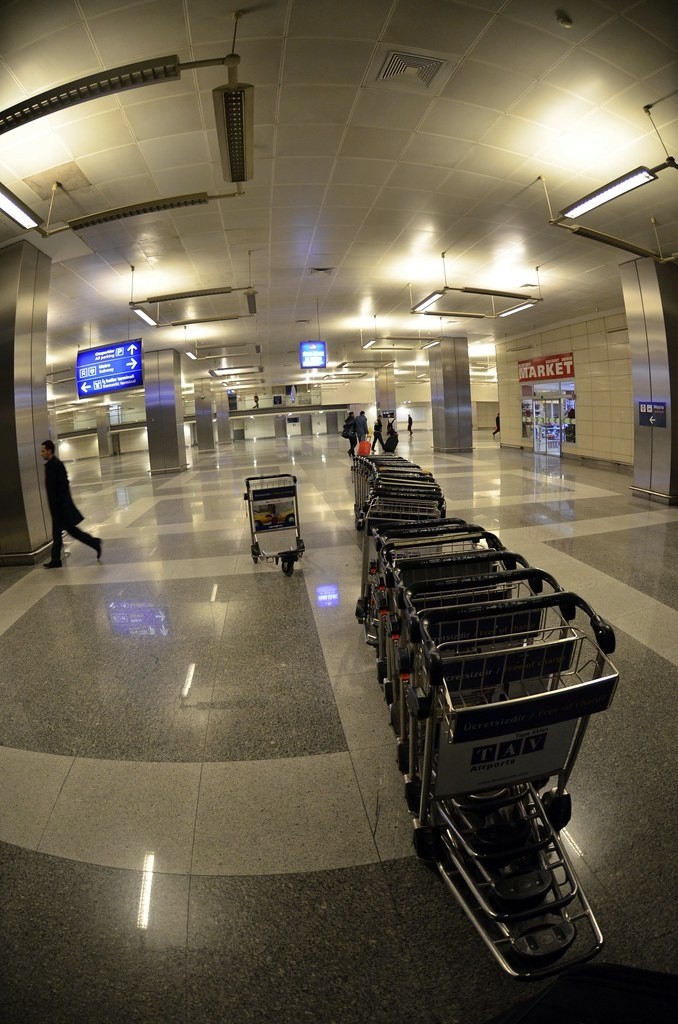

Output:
[387, 418, 397, 435]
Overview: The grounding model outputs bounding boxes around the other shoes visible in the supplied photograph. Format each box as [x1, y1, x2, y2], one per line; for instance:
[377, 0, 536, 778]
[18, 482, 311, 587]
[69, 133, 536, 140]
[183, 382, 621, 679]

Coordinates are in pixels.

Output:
[351, 452, 355, 457]
[348, 451, 351, 457]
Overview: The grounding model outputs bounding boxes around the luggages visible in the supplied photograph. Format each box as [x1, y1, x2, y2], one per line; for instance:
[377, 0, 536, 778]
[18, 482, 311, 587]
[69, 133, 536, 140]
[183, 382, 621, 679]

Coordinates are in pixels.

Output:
[357, 436, 371, 457]
[383, 437, 398, 454]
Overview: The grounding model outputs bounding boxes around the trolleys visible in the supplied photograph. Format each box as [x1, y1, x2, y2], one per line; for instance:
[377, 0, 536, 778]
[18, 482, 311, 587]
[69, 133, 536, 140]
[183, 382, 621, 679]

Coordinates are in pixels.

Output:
[348, 451, 619, 984]
[242, 472, 305, 577]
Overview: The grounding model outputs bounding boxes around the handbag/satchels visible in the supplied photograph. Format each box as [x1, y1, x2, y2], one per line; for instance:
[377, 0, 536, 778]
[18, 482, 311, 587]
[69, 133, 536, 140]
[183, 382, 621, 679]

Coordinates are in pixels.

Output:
[342, 428, 354, 438]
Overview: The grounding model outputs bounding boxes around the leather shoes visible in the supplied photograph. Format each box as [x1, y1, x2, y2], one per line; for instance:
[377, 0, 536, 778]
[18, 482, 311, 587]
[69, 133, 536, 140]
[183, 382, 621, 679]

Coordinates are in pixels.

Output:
[43, 560, 62, 568]
[94, 538, 101, 559]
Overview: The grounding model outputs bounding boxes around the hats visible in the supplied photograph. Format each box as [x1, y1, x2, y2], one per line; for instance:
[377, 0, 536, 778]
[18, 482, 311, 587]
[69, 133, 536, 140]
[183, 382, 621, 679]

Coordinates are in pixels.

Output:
[360, 411, 365, 415]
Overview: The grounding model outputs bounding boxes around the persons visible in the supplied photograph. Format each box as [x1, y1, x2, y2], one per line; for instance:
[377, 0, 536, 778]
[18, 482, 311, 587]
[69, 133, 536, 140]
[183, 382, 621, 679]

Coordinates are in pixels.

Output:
[41, 440, 102, 569]
[355, 411, 369, 443]
[387, 413, 399, 437]
[253, 393, 259, 408]
[371, 415, 385, 452]
[492, 413, 500, 435]
[407, 413, 413, 435]
[344, 411, 358, 457]
